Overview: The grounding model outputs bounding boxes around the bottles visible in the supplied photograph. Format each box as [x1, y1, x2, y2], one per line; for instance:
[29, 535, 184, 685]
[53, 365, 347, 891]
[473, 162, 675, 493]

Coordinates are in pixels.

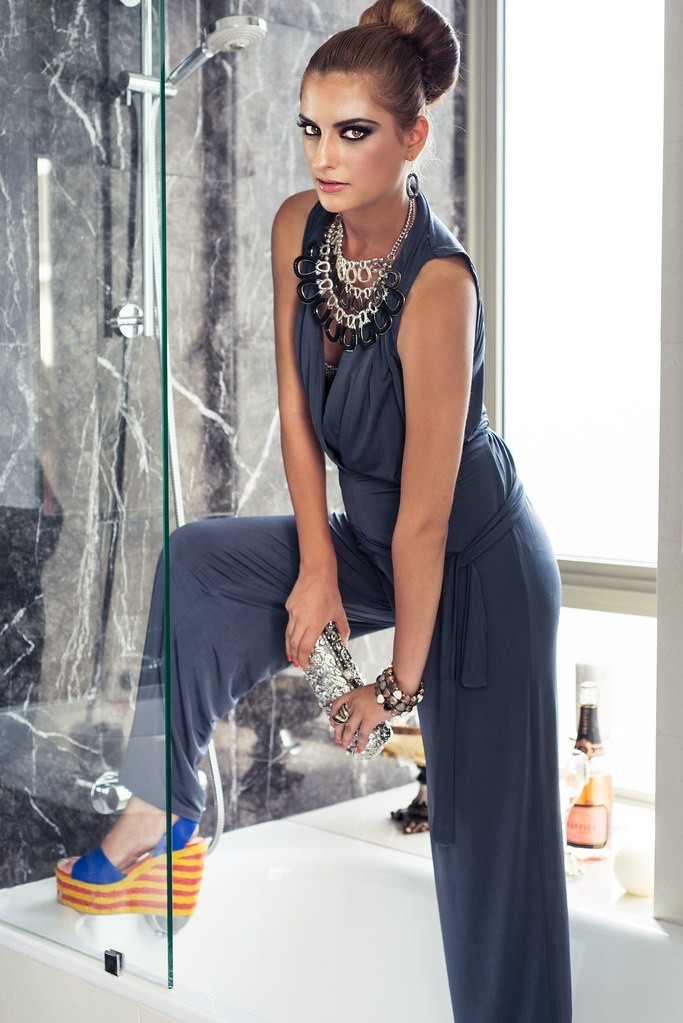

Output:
[567, 705, 612, 848]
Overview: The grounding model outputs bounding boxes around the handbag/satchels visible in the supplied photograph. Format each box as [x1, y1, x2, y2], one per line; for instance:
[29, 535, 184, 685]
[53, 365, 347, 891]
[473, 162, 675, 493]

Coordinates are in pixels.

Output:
[299, 621, 393, 764]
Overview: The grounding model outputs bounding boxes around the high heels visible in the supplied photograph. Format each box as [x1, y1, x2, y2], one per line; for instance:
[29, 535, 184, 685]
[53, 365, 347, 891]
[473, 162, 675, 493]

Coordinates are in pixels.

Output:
[54, 816, 205, 916]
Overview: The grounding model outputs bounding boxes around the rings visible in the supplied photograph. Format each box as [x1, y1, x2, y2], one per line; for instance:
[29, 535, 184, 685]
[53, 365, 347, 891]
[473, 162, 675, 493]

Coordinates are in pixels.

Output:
[332, 702, 352, 724]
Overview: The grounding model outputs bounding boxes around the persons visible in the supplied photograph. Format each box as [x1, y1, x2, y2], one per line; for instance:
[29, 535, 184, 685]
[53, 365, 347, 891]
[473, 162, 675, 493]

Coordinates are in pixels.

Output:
[54, 0, 571, 1023]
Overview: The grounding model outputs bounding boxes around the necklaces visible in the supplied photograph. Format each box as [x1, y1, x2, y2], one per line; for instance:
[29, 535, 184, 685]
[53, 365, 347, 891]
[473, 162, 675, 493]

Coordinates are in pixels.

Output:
[294, 198, 429, 352]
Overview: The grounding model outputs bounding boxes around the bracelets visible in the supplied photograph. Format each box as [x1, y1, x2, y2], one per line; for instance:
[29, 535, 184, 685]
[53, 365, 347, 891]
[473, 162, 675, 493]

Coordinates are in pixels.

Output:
[375, 667, 424, 716]
